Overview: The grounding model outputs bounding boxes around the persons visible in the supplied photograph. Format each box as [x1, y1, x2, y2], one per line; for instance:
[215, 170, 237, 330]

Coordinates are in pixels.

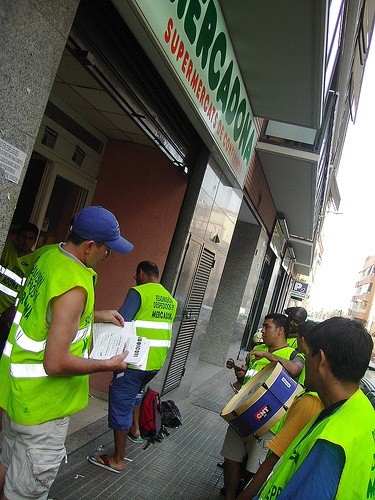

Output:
[0, 206, 134, 500]
[87, 261, 177, 472]
[0, 222, 39, 327]
[221, 307, 375, 500]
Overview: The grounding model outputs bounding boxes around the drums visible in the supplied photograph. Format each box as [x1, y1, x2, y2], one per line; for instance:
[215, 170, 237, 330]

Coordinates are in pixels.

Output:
[220, 361, 304, 443]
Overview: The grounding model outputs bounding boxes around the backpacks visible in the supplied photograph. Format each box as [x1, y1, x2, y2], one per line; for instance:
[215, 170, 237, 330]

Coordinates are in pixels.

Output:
[138, 387, 170, 450]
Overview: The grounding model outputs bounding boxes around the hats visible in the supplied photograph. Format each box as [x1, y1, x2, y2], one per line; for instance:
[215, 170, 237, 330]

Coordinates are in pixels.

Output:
[70, 206, 134, 254]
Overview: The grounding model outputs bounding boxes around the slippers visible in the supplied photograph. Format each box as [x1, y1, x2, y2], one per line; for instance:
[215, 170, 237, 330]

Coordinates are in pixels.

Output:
[128, 432, 142, 444]
[87, 455, 122, 473]
